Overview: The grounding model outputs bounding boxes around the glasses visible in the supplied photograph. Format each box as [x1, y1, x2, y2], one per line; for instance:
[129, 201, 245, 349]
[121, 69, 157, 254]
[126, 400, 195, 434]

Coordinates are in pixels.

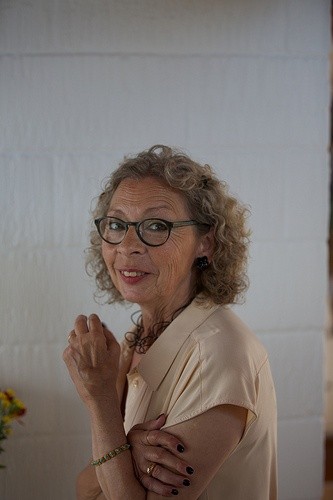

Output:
[93, 215, 206, 247]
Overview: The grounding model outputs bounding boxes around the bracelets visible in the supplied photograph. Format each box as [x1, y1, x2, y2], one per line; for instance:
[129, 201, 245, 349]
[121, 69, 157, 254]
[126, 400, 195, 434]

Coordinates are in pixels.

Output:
[91, 443, 131, 468]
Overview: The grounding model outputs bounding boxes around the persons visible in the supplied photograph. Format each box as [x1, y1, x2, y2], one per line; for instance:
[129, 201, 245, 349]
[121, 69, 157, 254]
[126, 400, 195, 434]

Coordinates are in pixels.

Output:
[62, 145, 279, 500]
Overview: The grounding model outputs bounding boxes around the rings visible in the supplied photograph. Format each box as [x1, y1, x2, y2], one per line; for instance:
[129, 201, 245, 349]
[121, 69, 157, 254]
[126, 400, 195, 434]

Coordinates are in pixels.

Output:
[146, 463, 158, 475]
[144, 430, 153, 446]
